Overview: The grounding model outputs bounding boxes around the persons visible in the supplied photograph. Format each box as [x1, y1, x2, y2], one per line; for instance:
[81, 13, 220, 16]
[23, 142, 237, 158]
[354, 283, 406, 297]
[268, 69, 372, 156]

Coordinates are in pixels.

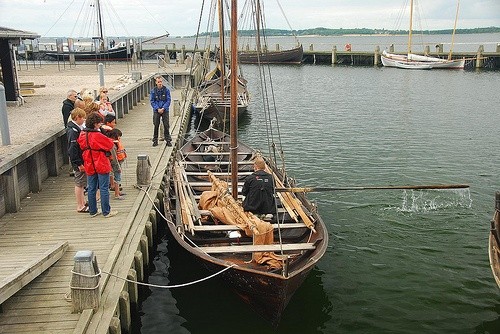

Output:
[66, 107, 91, 213]
[75, 86, 115, 116]
[108, 128, 128, 201]
[100, 113, 122, 192]
[239, 157, 277, 220]
[148, 75, 174, 148]
[61, 89, 78, 176]
[77, 112, 118, 216]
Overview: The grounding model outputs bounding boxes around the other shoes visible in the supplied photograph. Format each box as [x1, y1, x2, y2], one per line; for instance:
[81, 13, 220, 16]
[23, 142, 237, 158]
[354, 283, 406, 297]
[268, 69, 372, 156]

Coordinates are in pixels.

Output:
[153, 142, 158, 146]
[115, 196, 125, 200]
[90, 210, 102, 217]
[120, 192, 127, 195]
[109, 184, 122, 188]
[166, 141, 172, 146]
[110, 187, 122, 191]
[106, 210, 118, 217]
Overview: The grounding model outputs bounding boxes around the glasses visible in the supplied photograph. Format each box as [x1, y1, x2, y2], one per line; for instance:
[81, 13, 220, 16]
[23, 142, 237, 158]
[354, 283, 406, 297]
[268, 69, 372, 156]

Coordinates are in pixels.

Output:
[101, 90, 108, 94]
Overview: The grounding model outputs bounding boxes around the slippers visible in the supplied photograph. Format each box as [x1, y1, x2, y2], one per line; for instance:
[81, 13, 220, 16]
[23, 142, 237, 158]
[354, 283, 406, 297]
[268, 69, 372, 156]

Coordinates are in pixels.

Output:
[78, 205, 90, 212]
[84, 203, 88, 206]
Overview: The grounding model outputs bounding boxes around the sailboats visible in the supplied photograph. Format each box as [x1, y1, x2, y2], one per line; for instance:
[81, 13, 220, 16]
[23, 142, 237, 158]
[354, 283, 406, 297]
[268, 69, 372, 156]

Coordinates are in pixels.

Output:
[159, 0, 330, 332]
[213, 0, 304, 65]
[182, 0, 249, 119]
[16, 32, 170, 59]
[380, 50, 466, 71]
